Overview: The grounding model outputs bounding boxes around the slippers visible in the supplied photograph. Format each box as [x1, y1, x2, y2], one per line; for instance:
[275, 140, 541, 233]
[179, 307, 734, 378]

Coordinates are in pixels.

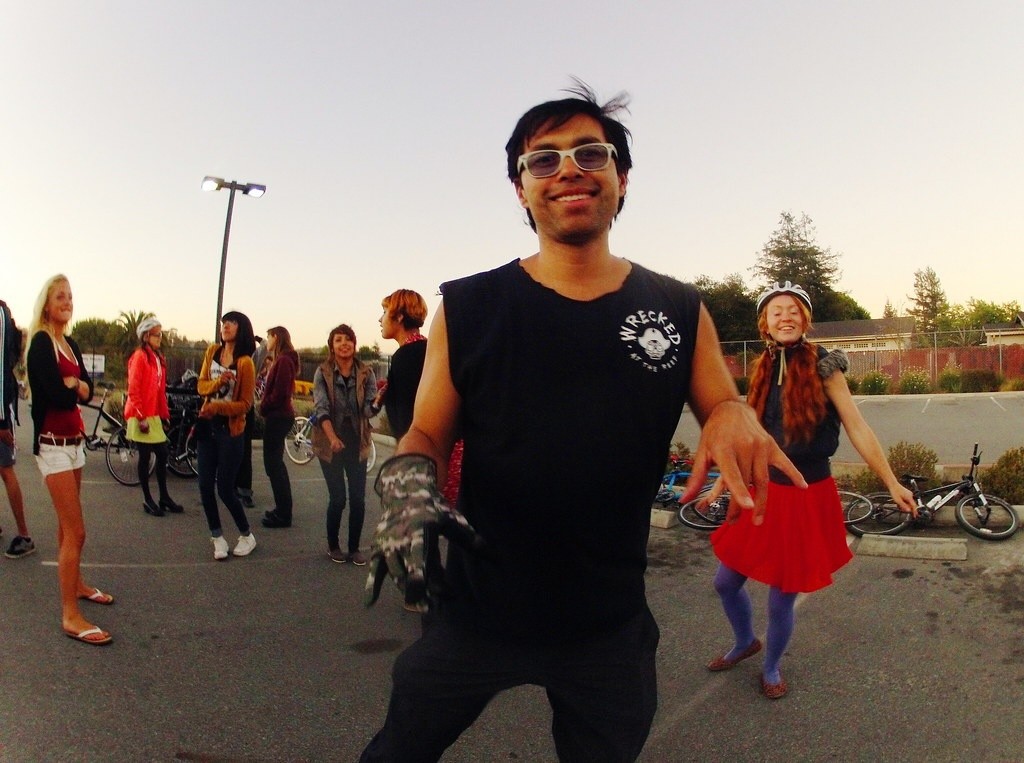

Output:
[63, 625, 112, 646]
[79, 588, 114, 604]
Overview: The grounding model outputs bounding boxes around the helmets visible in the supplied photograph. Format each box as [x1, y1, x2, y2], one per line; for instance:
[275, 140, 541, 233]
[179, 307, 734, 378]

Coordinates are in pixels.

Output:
[136, 316, 161, 340]
[756, 280, 813, 318]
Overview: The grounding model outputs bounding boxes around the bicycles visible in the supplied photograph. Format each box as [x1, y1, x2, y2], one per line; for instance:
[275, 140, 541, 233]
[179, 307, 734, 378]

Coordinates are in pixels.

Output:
[286, 407, 377, 473]
[79, 380, 206, 485]
[651, 444, 1018, 544]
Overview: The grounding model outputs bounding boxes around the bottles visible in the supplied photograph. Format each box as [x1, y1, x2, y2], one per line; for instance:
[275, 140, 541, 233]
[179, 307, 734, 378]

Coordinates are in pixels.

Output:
[926, 494, 943, 509]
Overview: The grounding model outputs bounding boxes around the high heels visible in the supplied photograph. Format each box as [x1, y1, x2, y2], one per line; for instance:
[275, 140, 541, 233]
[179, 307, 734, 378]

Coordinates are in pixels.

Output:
[159, 497, 184, 514]
[143, 501, 164, 516]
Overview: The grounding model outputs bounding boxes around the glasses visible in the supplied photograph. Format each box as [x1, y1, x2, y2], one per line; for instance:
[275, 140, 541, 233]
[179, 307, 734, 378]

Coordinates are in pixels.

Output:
[149, 331, 162, 338]
[517, 143, 619, 178]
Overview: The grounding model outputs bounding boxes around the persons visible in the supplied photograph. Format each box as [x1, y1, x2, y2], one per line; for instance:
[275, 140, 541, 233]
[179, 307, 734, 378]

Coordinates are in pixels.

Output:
[196, 312, 272, 559]
[311, 323, 388, 566]
[125, 317, 185, 517]
[257, 326, 303, 529]
[696, 279, 917, 698]
[0, 301, 36, 558]
[356, 73, 807, 763]
[23, 274, 114, 646]
[378, 289, 462, 611]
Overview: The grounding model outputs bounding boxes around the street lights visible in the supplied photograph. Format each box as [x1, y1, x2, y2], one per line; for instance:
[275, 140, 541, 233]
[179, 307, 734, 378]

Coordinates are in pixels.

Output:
[201, 176, 266, 346]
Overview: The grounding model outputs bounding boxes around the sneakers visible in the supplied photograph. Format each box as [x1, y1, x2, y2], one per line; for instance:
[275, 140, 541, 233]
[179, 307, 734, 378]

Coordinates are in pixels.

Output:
[234, 532, 257, 556]
[210, 535, 229, 559]
[5, 535, 36, 558]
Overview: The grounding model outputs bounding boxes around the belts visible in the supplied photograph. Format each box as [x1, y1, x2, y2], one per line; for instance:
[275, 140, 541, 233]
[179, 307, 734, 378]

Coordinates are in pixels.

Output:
[40, 435, 82, 447]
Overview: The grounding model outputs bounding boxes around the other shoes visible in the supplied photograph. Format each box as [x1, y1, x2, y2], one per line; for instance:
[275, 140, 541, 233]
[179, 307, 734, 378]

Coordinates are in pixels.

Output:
[326, 546, 347, 563]
[264, 510, 278, 519]
[262, 518, 286, 528]
[763, 671, 787, 698]
[708, 638, 761, 671]
[350, 552, 366, 566]
[240, 495, 254, 509]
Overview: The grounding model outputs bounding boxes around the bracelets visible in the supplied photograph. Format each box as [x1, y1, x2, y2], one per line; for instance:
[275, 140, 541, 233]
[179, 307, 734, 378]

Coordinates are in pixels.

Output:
[76, 379, 80, 389]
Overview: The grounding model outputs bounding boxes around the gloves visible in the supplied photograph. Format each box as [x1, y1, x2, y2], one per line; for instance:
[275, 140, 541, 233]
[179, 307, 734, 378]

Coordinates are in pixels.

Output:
[362, 455, 476, 613]
